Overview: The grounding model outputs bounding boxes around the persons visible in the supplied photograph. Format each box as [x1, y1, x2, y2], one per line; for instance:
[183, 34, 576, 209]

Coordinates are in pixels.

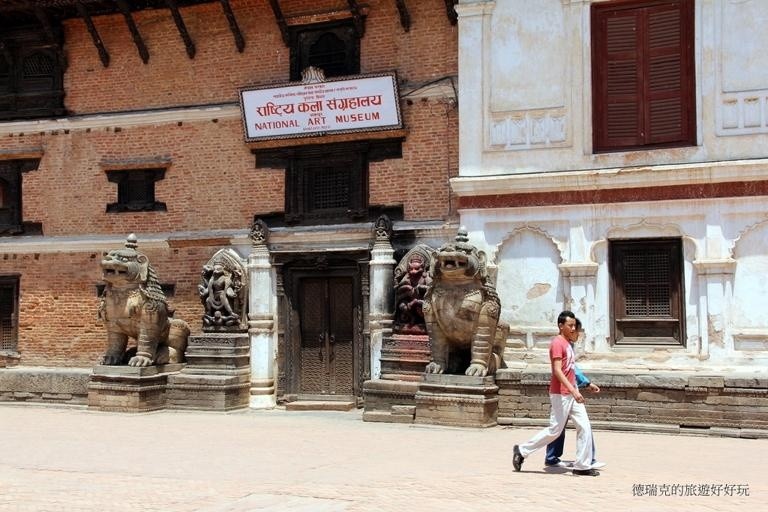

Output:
[544, 316, 607, 470]
[512, 311, 601, 476]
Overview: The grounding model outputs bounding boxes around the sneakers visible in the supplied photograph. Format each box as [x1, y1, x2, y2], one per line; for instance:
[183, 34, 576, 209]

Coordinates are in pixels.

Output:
[512, 443, 523, 472]
[572, 468, 601, 476]
[591, 461, 606, 469]
[544, 459, 574, 468]
